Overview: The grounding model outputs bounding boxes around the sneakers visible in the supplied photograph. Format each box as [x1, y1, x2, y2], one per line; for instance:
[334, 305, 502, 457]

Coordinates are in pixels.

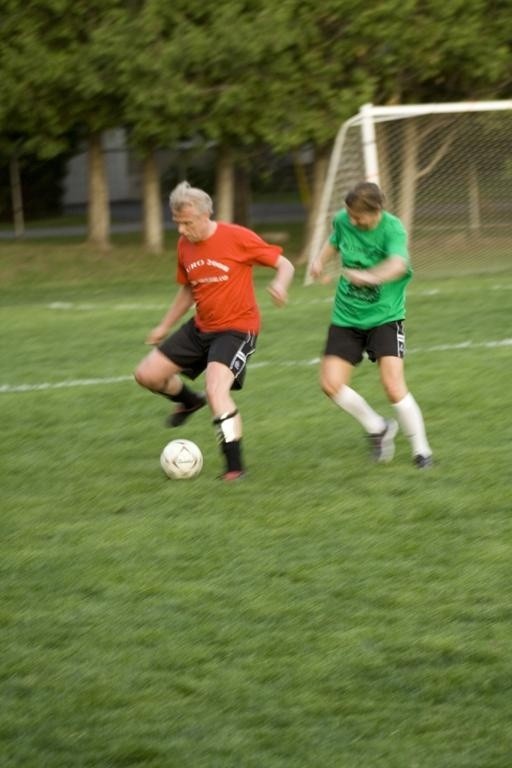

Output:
[412, 448, 434, 469]
[214, 467, 245, 482]
[365, 417, 400, 464]
[163, 391, 206, 430]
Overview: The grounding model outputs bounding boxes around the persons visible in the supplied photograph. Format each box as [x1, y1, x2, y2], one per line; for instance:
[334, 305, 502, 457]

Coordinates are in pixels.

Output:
[134, 179, 297, 478]
[311, 182, 433, 471]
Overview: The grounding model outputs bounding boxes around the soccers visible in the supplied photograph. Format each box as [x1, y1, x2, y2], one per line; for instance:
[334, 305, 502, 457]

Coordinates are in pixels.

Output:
[159, 438, 202, 480]
